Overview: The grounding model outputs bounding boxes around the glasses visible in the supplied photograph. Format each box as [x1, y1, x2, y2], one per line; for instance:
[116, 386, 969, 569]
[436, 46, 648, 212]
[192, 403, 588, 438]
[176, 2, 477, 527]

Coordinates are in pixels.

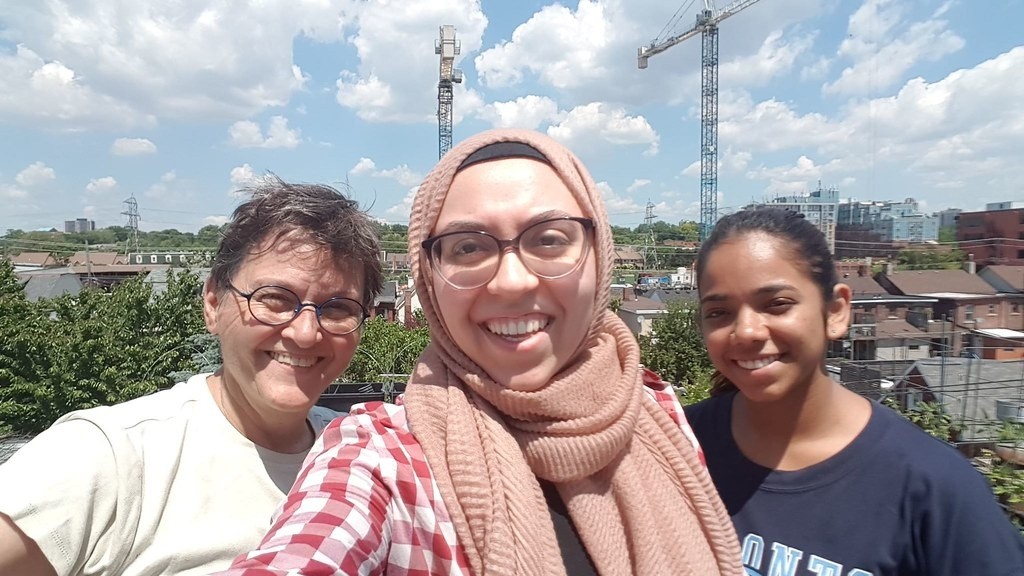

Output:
[224, 281, 370, 335]
[421, 217, 596, 290]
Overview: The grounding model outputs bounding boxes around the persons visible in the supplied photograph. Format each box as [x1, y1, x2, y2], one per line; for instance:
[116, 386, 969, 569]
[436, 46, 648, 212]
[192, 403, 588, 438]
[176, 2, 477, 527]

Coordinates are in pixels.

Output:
[242, 127, 749, 576]
[0, 168, 383, 576]
[683, 204, 1024, 576]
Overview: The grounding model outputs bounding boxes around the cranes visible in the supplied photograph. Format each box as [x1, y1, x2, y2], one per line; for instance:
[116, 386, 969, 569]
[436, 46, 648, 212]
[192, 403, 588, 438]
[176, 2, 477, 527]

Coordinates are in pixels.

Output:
[435, 24, 463, 159]
[638, 0, 759, 242]
